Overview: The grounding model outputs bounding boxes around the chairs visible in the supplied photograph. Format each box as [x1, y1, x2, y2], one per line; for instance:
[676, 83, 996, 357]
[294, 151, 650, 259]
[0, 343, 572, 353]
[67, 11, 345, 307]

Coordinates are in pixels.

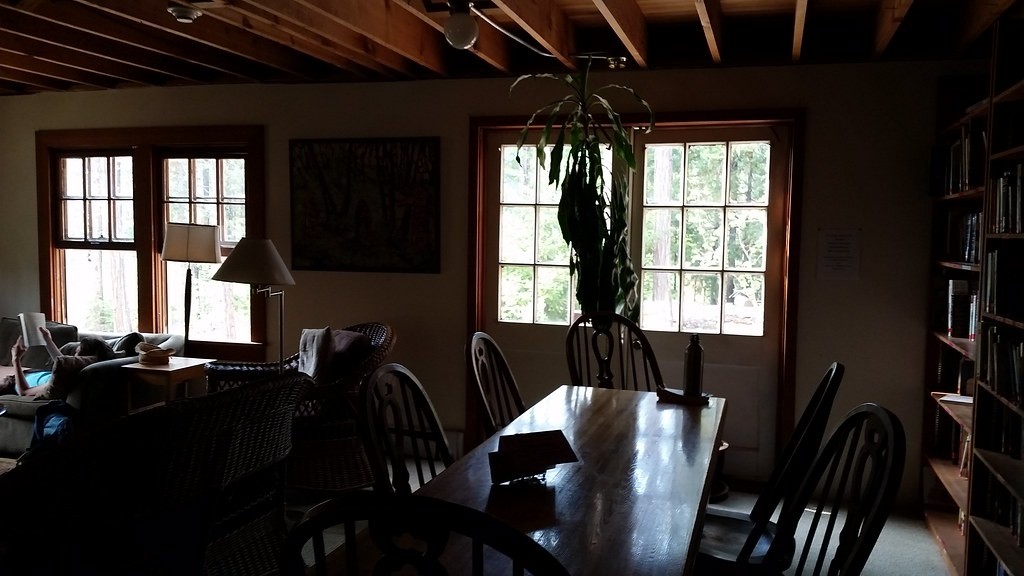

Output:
[565, 311, 666, 394]
[204, 320, 398, 420]
[277, 487, 573, 576]
[696, 360, 848, 576]
[758, 401, 906, 576]
[350, 360, 455, 512]
[467, 331, 528, 444]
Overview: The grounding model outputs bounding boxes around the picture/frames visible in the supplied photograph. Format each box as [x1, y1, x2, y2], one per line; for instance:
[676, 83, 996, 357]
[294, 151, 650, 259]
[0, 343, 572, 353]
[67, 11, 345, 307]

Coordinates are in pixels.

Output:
[288, 135, 442, 275]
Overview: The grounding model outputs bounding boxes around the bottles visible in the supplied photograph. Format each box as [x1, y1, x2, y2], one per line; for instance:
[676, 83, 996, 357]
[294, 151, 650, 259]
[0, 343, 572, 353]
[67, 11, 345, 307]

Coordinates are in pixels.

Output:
[682, 333, 704, 396]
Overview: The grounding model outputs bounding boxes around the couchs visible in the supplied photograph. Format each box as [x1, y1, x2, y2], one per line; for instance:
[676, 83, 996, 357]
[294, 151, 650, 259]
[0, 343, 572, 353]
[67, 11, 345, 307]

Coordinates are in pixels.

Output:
[1, 316, 187, 462]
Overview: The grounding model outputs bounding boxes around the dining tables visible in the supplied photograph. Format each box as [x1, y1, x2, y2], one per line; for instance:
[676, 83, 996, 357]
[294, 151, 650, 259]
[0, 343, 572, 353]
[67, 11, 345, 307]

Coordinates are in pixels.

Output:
[300, 381, 728, 576]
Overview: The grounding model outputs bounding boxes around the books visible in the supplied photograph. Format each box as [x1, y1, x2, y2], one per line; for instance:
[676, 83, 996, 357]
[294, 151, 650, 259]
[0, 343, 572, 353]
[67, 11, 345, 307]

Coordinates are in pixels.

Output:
[18, 312, 49, 347]
[934, 130, 1023, 576]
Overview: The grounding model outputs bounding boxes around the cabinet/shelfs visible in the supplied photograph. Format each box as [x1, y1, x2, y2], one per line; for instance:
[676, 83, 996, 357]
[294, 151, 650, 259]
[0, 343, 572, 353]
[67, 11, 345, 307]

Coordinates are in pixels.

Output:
[916, 80, 1024, 576]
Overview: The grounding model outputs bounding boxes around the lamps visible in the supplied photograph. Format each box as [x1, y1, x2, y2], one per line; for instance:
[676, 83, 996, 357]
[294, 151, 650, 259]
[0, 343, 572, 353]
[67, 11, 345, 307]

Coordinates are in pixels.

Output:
[211, 236, 296, 375]
[444, 0, 480, 51]
[162, 222, 222, 358]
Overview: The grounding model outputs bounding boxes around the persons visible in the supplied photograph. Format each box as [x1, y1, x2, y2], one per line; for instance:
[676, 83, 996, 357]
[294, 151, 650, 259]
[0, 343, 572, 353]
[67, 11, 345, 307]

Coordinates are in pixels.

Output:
[12, 328, 115, 396]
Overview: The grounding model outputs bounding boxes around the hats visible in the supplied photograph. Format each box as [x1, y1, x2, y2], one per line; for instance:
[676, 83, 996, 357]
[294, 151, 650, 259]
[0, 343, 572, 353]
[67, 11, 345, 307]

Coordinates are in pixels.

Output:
[135, 341, 176, 365]
